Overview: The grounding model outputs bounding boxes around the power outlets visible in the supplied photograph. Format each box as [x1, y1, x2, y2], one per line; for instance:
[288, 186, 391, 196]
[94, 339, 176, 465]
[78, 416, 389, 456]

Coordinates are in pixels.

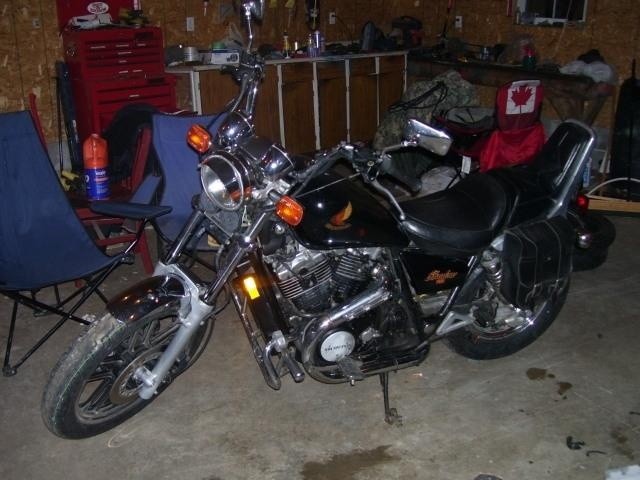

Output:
[328, 11, 337, 24]
[454, 15, 464, 28]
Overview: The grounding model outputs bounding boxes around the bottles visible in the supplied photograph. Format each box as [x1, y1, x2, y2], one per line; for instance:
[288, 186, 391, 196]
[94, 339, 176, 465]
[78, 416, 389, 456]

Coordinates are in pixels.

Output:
[282, 29, 324, 60]
[361, 20, 389, 52]
[184, 40, 225, 66]
[523, 50, 537, 70]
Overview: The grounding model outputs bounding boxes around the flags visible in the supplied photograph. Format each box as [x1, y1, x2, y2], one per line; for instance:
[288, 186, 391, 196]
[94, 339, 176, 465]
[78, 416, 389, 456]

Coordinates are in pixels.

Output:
[496, 79, 544, 130]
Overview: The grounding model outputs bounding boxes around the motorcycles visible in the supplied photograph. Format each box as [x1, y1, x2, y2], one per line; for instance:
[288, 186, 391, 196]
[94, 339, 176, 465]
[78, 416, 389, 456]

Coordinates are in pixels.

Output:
[42, 0, 619, 440]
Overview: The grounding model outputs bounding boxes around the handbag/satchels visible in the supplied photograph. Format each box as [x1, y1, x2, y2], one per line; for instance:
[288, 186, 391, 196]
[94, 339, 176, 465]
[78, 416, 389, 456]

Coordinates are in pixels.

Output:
[372, 68, 479, 150]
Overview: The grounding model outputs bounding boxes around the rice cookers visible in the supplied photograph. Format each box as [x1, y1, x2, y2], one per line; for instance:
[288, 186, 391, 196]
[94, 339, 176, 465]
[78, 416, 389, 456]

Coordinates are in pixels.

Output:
[391, 15, 424, 51]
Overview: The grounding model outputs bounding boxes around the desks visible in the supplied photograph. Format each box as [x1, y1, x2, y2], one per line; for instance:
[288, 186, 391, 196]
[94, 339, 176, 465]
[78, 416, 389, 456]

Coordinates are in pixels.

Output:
[163, 38, 410, 150]
[410, 46, 618, 127]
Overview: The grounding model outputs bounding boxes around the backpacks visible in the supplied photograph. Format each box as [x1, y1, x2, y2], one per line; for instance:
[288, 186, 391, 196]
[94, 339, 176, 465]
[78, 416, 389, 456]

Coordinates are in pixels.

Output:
[106, 102, 161, 183]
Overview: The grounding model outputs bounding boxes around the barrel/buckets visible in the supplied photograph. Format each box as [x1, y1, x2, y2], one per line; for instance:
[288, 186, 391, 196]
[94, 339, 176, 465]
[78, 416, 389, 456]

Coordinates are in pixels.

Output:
[583, 155, 593, 190]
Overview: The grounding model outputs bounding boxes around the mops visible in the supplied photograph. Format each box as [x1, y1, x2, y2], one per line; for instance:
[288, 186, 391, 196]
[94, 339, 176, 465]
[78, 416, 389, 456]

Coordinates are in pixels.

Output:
[587, 56, 640, 215]
